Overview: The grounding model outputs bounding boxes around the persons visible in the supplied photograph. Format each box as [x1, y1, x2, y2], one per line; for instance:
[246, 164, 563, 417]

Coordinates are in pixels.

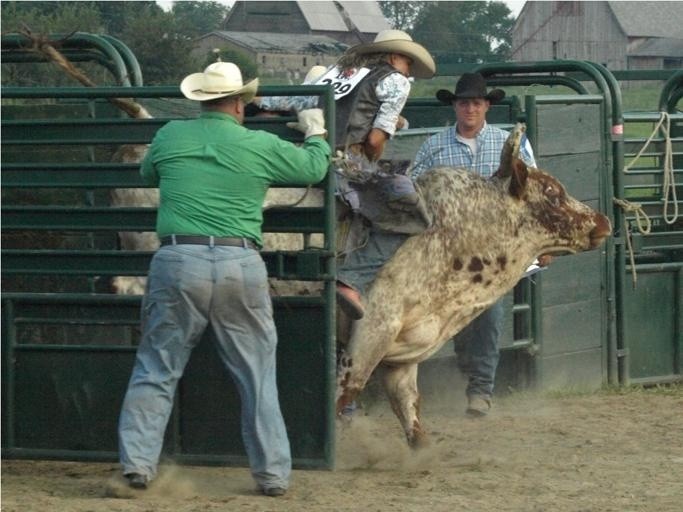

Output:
[248, 63, 410, 132]
[116, 62, 334, 498]
[408, 70, 554, 423]
[307, 29, 435, 320]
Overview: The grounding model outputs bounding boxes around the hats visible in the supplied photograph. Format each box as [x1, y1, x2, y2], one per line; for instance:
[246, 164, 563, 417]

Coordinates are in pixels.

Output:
[179, 62, 258, 104]
[436, 72, 505, 106]
[301, 66, 327, 85]
[346, 30, 436, 79]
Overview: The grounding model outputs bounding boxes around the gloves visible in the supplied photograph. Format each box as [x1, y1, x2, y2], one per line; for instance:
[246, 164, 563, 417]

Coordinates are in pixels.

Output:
[286, 108, 328, 139]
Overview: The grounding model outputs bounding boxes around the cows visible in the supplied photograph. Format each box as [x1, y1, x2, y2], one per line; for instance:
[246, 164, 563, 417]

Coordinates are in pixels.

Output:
[15, 18, 614, 454]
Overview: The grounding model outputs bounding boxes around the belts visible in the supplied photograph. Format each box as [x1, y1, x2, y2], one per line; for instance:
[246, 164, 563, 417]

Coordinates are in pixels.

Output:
[161, 236, 255, 249]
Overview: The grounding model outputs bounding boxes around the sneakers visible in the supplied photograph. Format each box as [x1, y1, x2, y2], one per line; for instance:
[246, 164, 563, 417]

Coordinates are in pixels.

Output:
[336, 282, 365, 321]
[264, 487, 285, 496]
[130, 472, 148, 489]
[465, 391, 490, 415]
